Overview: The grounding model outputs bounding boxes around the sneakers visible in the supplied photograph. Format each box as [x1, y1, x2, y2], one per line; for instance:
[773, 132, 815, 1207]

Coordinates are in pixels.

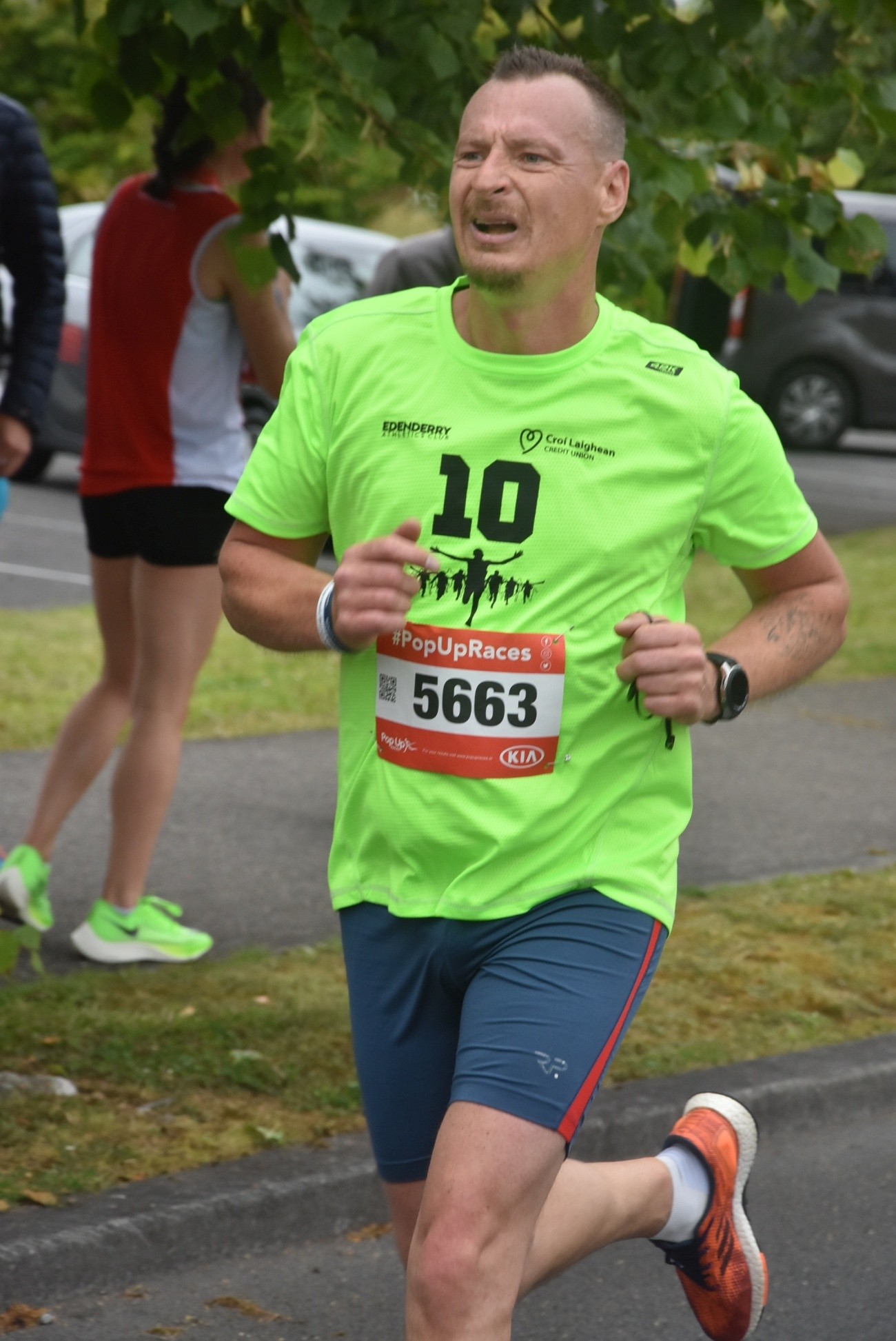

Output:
[651, 1092, 770, 1341]
[70, 894, 214, 964]
[1, 845, 54, 932]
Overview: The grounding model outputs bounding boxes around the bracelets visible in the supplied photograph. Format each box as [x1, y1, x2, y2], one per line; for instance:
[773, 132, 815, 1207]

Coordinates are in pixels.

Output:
[323, 592, 361, 656]
[316, 580, 335, 649]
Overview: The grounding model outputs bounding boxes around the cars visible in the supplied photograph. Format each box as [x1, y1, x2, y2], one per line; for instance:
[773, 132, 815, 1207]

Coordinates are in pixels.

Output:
[0, 197, 407, 483]
[670, 160, 895, 453]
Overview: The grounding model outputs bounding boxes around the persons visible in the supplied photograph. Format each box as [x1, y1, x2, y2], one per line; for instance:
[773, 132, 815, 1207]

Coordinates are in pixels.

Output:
[220, 49, 854, 1341]
[0, 93, 298, 964]
[368, 222, 467, 297]
[0, 96, 68, 515]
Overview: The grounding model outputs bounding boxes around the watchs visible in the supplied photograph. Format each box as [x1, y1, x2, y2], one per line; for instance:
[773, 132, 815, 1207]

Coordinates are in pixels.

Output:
[705, 649, 750, 727]
